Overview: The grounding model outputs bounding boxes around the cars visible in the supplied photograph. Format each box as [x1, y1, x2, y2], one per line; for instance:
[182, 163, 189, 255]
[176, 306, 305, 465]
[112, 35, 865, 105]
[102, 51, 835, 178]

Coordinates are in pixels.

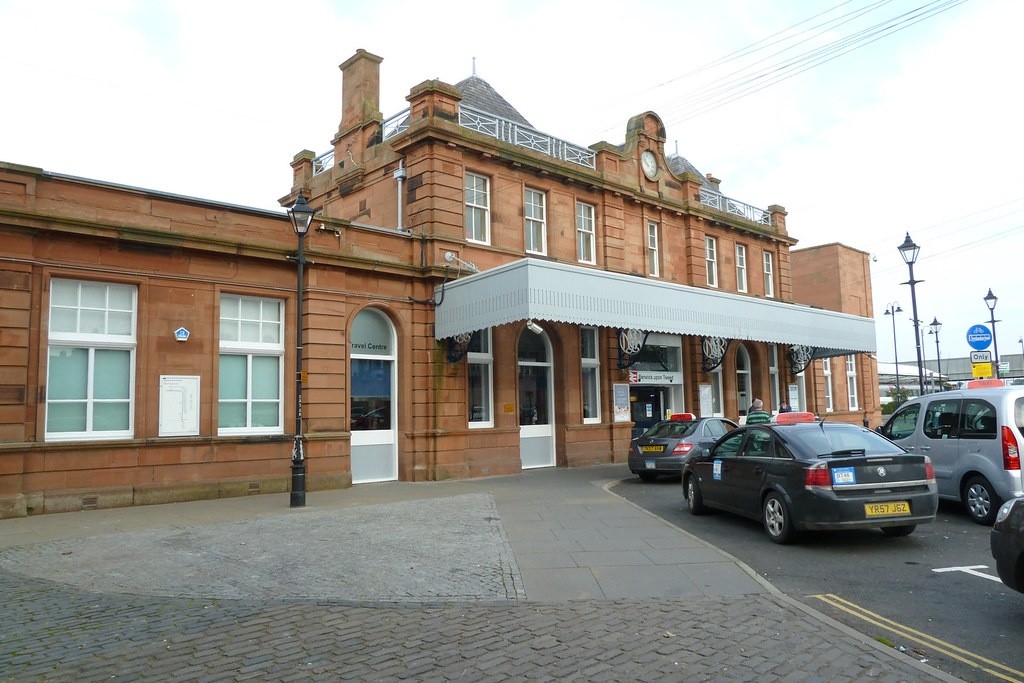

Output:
[355, 408, 387, 430]
[470, 406, 486, 421]
[628, 413, 741, 482]
[681, 412, 939, 544]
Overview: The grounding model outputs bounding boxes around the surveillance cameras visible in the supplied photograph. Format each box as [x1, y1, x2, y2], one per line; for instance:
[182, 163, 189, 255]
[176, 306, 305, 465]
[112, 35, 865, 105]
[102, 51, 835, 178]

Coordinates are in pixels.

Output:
[789, 345, 799, 353]
[527, 323, 543, 334]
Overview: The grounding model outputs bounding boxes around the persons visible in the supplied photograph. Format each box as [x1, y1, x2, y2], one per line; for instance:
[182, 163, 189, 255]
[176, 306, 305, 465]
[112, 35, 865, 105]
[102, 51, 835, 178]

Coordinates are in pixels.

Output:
[747, 399, 773, 443]
[779, 401, 792, 412]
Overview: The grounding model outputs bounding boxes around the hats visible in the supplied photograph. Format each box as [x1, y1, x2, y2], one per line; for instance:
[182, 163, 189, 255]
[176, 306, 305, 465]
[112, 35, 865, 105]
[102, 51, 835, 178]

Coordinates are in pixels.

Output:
[752, 399, 763, 407]
[778, 401, 786, 405]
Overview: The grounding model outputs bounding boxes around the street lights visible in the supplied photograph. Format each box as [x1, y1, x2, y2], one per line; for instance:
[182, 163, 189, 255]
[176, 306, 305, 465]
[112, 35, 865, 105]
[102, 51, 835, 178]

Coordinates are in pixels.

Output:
[286, 188, 315, 507]
[883, 301, 903, 394]
[930, 316, 942, 392]
[921, 326, 934, 394]
[897, 231, 926, 396]
[983, 288, 1002, 379]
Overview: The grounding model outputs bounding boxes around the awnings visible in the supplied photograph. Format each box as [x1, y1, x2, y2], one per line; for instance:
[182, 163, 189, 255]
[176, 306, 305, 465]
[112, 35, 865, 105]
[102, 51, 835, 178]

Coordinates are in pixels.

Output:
[434, 258, 876, 360]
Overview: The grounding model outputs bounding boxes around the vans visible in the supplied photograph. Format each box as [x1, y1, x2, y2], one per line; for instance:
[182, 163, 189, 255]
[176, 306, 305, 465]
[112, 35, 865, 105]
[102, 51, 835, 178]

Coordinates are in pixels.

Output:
[873, 385, 1024, 525]
